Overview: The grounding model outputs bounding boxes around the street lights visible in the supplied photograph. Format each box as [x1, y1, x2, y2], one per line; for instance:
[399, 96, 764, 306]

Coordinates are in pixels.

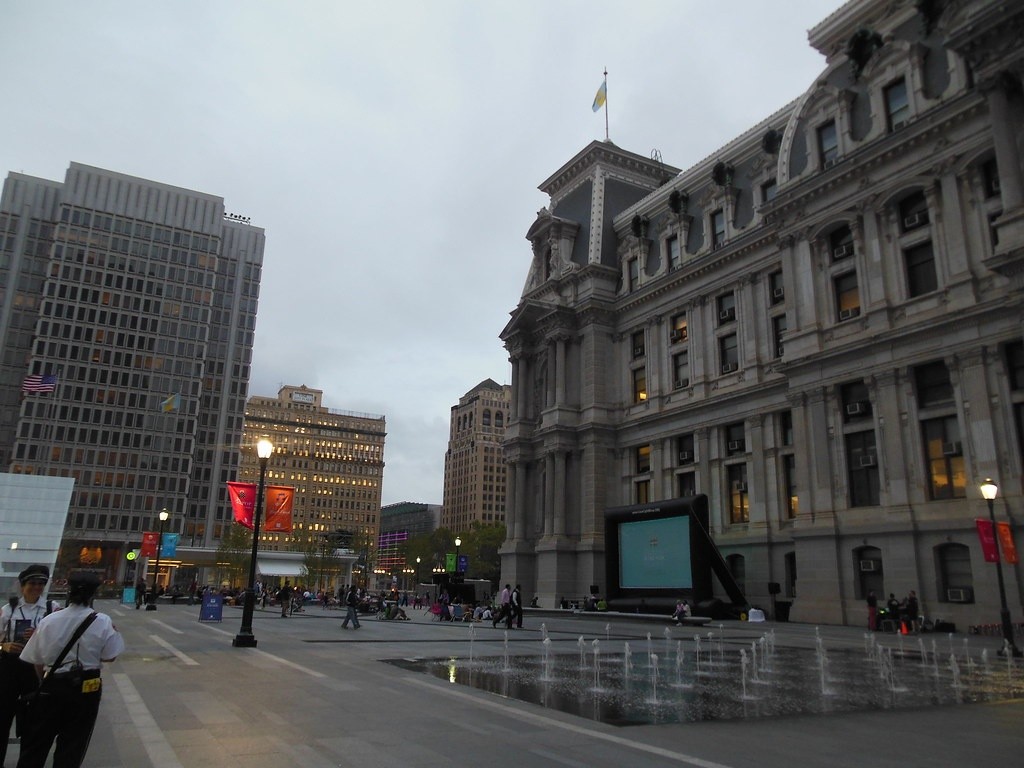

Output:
[433, 564, 446, 585]
[402, 564, 415, 591]
[455, 537, 461, 603]
[374, 566, 386, 595]
[146, 509, 169, 611]
[416, 556, 420, 585]
[232, 437, 273, 645]
[981, 478, 1022, 658]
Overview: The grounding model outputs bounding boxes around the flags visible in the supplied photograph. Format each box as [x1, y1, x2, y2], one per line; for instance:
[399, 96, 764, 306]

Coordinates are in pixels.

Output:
[591, 81, 605, 112]
[20, 374, 56, 392]
[159, 389, 183, 416]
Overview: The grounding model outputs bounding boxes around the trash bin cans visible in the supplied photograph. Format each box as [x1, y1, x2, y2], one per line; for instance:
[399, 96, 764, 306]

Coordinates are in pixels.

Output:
[386, 601, 397, 618]
[775, 601, 792, 622]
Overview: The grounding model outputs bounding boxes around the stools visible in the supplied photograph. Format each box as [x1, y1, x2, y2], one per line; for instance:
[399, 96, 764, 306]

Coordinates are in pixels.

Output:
[881, 619, 896, 632]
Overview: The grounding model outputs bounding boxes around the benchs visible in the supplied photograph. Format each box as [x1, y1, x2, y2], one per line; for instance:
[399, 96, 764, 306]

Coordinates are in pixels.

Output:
[159, 595, 233, 604]
[257, 597, 396, 607]
[521, 607, 712, 627]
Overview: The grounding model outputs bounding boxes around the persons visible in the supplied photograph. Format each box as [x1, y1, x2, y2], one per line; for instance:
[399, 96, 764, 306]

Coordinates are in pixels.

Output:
[866, 586, 880, 633]
[0, 564, 59, 768]
[136, 576, 146, 611]
[584, 594, 609, 612]
[672, 598, 691, 628]
[154, 578, 523, 630]
[885, 588, 918, 633]
[22, 571, 124, 768]
[530, 596, 538, 608]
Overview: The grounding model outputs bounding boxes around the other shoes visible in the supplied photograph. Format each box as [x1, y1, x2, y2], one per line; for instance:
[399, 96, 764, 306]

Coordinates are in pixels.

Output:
[505, 619, 508, 627]
[282, 614, 288, 617]
[354, 624, 360, 630]
[676, 623, 682, 627]
[493, 621, 496, 628]
[517, 626, 524, 628]
[341, 624, 348, 629]
[508, 628, 514, 629]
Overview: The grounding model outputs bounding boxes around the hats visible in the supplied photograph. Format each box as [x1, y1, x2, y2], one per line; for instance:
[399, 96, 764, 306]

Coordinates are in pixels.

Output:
[68, 571, 102, 591]
[18, 565, 49, 585]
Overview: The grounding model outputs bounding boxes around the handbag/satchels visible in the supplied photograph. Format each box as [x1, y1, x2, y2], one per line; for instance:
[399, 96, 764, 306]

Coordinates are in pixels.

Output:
[16, 690, 41, 737]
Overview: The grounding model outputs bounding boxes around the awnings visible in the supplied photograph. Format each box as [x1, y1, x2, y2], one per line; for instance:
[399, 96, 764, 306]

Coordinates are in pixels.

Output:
[255, 558, 312, 576]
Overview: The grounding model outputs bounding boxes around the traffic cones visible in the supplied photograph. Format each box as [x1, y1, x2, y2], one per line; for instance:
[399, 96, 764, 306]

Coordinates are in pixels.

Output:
[900, 621, 908, 635]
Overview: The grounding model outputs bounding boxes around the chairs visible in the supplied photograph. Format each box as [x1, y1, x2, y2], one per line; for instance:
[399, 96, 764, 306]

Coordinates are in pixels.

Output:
[453, 613, 468, 623]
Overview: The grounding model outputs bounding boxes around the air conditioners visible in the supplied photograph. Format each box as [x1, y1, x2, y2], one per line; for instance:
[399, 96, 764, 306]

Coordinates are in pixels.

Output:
[675, 379, 686, 388]
[779, 346, 784, 356]
[992, 178, 1001, 191]
[825, 159, 835, 170]
[943, 441, 962, 454]
[847, 402, 863, 414]
[723, 363, 735, 373]
[834, 245, 846, 258]
[861, 455, 876, 466]
[680, 452, 690, 460]
[774, 288, 783, 298]
[729, 441, 740, 450]
[948, 589, 969, 601]
[634, 348, 642, 354]
[861, 559, 876, 571]
[904, 214, 920, 228]
[839, 308, 856, 320]
[720, 309, 730, 319]
[670, 329, 681, 339]
[685, 490, 693, 496]
[736, 482, 748, 490]
[714, 242, 723, 250]
